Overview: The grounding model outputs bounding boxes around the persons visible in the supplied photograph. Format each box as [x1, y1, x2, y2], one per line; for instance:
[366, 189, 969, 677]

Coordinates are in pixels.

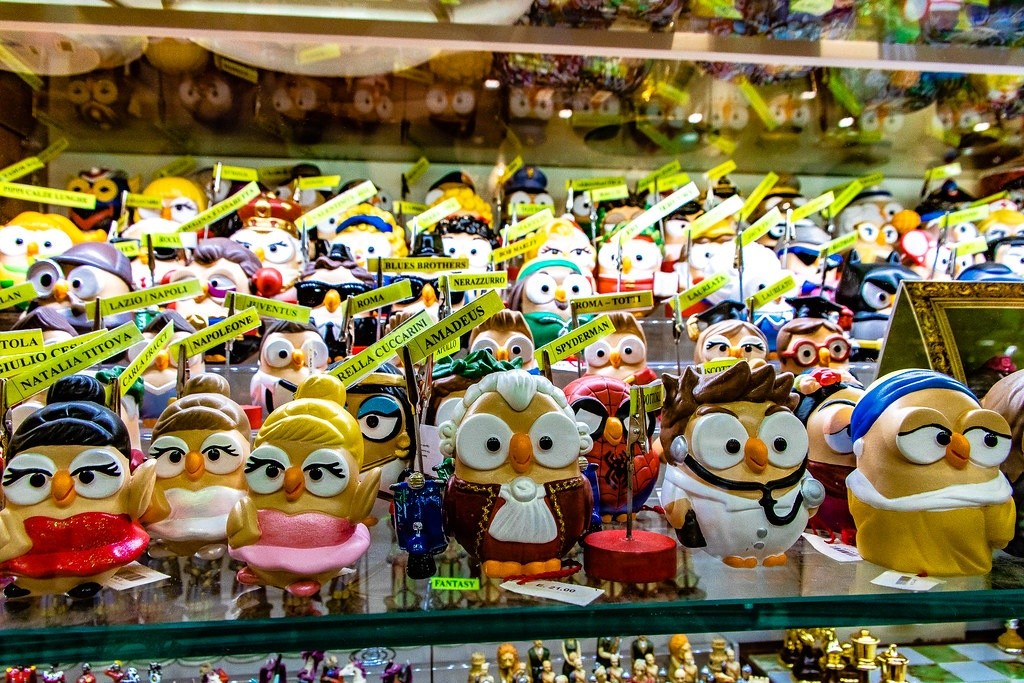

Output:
[0, 636, 766, 683]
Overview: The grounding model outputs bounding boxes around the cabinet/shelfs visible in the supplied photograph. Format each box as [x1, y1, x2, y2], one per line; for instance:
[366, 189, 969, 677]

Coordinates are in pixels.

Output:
[0, 0, 1024, 683]
